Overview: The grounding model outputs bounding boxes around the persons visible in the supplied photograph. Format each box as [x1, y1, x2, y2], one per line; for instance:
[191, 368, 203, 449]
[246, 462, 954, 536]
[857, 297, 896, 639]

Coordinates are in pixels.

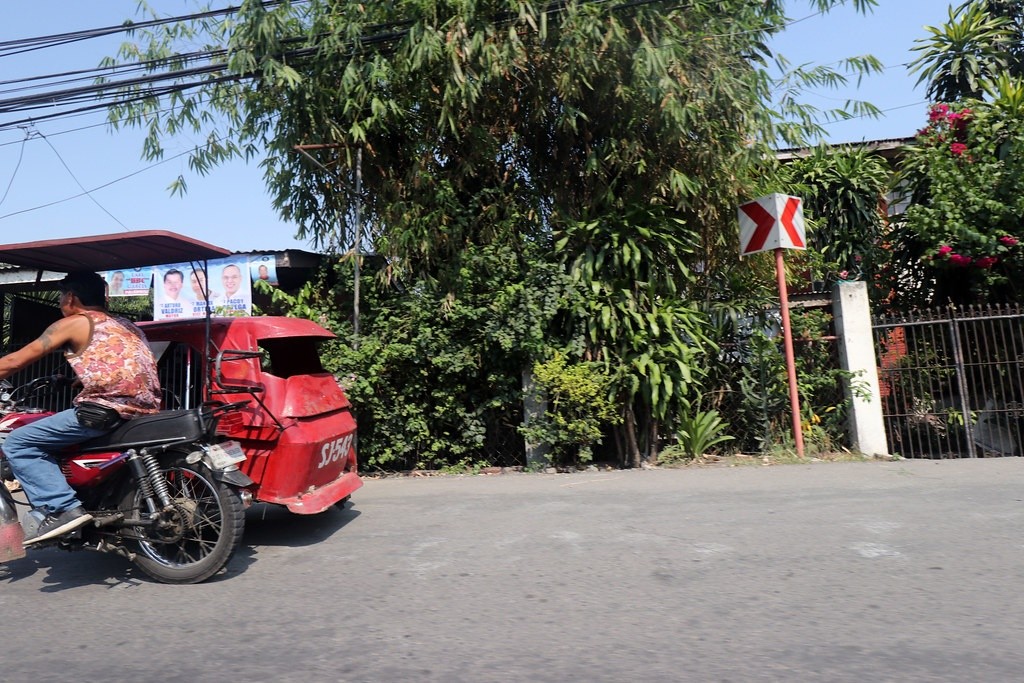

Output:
[189, 268, 220, 304]
[214, 264, 251, 312]
[254, 265, 277, 284]
[162, 269, 192, 312]
[0, 270, 163, 544]
[106, 271, 124, 296]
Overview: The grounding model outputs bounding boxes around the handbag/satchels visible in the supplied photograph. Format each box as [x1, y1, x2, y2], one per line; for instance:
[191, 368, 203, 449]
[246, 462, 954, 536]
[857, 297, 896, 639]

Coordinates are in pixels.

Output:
[76, 401, 122, 430]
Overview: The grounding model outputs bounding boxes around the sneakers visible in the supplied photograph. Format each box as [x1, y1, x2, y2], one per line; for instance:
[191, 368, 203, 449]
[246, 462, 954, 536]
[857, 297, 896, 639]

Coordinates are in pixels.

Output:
[22, 506, 93, 545]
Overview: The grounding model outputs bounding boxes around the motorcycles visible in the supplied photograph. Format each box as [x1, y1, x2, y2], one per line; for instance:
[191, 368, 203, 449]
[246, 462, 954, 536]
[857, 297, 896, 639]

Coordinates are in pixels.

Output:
[0, 230, 363, 585]
